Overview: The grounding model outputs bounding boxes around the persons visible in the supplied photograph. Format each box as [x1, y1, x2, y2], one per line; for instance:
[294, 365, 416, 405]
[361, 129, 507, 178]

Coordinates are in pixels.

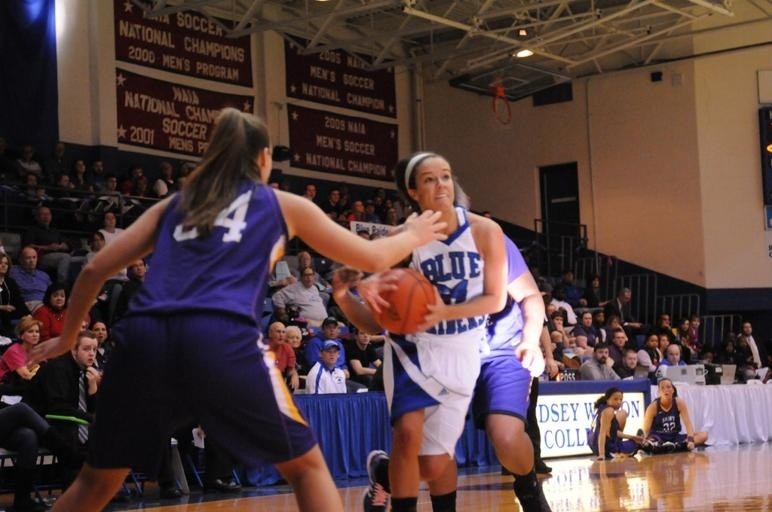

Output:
[2, 105, 772, 512]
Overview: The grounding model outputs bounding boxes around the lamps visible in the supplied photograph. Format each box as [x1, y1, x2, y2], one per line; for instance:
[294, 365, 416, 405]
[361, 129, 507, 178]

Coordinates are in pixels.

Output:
[511, 29, 533, 58]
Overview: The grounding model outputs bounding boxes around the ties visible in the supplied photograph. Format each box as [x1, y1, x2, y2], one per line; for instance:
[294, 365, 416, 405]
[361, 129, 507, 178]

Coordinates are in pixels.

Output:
[76, 373, 89, 447]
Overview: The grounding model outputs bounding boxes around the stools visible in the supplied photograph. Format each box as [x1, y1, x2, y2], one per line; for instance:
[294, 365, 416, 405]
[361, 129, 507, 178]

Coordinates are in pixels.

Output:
[0, 444, 241, 512]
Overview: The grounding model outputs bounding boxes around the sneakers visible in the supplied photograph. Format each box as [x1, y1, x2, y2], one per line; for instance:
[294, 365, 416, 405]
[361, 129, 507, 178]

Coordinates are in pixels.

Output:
[512, 476, 553, 512]
[361, 448, 392, 511]
[654, 440, 687, 453]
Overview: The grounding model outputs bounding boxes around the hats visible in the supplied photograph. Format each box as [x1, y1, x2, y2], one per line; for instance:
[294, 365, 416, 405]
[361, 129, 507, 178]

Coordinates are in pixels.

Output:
[318, 338, 342, 352]
[322, 315, 339, 329]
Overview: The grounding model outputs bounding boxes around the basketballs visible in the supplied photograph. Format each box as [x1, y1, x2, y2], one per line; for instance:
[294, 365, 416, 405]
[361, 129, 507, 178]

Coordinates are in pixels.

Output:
[372, 268, 435, 334]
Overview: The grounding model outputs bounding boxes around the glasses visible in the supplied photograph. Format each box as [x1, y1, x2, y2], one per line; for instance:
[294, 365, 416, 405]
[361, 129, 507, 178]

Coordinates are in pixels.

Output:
[303, 273, 315, 276]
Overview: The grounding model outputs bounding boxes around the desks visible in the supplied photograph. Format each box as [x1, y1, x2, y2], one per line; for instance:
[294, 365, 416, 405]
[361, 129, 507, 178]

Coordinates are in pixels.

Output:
[234, 380, 650, 487]
[651, 383, 772, 445]
